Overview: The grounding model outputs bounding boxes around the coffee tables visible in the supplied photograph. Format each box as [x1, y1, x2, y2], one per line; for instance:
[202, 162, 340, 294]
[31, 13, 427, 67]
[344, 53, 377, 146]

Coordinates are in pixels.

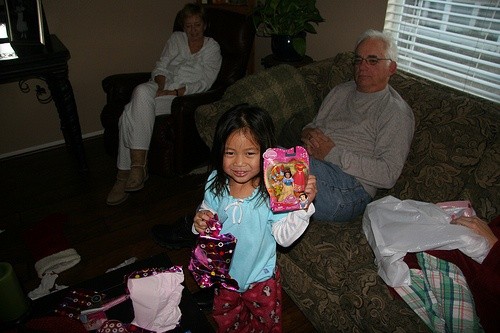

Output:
[28, 252, 216, 333]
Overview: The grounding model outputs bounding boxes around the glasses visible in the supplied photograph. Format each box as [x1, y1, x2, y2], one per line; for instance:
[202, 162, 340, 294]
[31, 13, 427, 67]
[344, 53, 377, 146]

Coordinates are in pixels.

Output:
[351, 56, 393, 66]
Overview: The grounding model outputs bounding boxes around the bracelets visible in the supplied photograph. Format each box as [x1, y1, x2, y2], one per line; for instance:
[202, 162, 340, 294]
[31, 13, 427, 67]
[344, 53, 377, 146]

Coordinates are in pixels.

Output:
[174, 89, 178, 96]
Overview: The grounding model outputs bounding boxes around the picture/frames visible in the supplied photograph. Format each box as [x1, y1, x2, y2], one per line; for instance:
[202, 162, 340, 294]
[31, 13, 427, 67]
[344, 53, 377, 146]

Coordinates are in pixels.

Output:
[4, 0, 45, 46]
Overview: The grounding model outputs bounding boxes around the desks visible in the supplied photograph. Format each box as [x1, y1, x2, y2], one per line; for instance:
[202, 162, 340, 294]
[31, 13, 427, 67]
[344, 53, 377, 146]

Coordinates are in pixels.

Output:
[0, 34, 90, 178]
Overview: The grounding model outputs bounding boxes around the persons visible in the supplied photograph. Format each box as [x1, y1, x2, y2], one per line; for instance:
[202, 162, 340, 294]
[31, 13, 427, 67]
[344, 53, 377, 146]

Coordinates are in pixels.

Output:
[191, 103, 318, 333]
[107, 4, 221, 206]
[302, 30, 416, 222]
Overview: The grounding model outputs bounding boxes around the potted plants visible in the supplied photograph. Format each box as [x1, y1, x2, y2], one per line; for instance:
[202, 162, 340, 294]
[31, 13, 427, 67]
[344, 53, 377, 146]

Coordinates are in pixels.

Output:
[251, 0, 327, 63]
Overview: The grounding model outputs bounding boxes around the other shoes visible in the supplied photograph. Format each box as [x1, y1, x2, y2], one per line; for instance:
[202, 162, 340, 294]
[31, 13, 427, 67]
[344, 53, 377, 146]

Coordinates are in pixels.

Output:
[123, 161, 149, 192]
[106, 176, 129, 204]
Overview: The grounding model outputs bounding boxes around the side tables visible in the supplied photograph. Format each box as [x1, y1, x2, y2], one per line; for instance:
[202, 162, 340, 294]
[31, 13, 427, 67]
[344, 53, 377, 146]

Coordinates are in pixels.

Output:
[260, 54, 313, 69]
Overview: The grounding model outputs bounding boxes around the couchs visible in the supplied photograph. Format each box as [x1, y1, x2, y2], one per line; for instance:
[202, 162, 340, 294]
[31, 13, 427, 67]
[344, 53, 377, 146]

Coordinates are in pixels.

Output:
[100, 7, 256, 181]
[193, 51, 500, 333]
